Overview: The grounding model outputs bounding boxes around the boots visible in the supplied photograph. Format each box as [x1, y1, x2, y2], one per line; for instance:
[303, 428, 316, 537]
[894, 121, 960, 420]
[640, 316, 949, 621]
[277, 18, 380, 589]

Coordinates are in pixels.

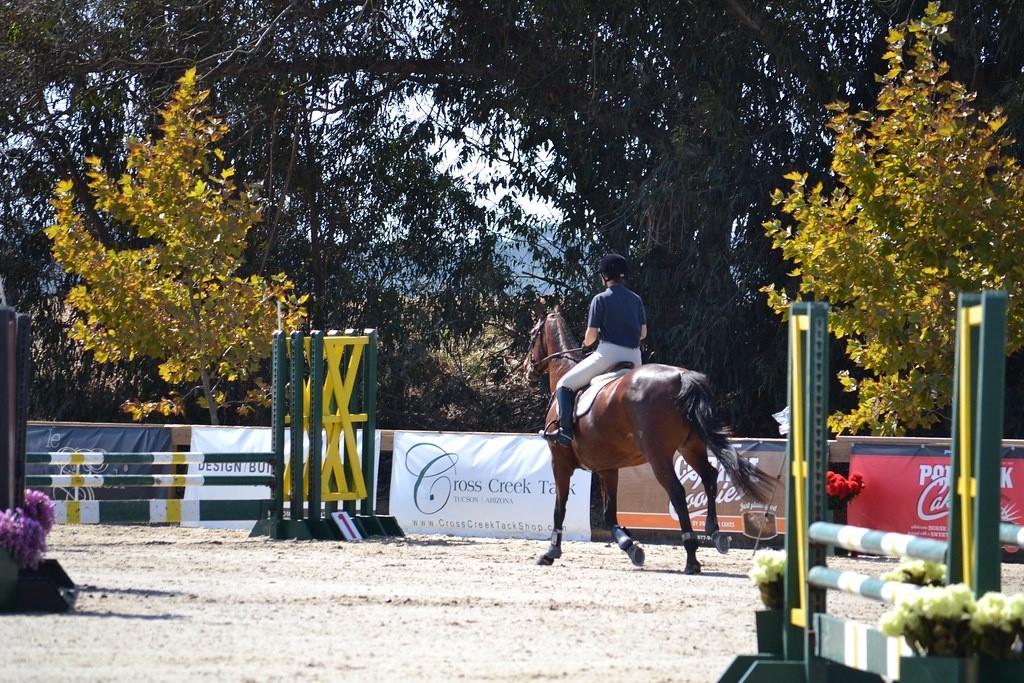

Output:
[538, 387, 576, 449]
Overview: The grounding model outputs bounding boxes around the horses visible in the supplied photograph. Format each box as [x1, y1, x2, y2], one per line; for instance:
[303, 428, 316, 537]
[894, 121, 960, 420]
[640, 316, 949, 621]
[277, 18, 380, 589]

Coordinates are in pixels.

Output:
[522, 300, 786, 576]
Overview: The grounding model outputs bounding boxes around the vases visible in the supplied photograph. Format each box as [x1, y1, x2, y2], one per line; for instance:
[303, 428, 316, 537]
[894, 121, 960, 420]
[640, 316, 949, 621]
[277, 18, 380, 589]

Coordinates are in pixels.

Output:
[904, 622, 971, 658]
[976, 630, 1017, 659]
[758, 582, 785, 609]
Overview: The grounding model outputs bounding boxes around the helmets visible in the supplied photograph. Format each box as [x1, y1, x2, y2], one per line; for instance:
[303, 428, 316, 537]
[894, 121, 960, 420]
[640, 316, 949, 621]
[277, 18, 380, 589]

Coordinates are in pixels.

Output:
[594, 253, 631, 278]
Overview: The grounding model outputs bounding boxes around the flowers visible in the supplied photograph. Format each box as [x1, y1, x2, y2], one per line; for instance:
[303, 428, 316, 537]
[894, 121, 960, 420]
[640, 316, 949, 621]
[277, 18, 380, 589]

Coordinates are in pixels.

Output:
[0, 488, 57, 570]
[747, 549, 787, 586]
[879, 559, 1024, 658]
[827, 470, 865, 510]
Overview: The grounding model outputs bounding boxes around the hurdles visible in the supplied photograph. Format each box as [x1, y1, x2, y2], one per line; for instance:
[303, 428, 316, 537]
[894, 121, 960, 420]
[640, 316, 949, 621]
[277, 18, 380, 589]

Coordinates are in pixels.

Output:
[712, 288, 1024, 682]
[0, 301, 408, 541]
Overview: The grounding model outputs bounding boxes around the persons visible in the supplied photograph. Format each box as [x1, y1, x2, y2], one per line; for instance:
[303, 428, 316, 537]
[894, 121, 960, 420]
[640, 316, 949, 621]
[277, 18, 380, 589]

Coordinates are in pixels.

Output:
[538, 254, 647, 449]
